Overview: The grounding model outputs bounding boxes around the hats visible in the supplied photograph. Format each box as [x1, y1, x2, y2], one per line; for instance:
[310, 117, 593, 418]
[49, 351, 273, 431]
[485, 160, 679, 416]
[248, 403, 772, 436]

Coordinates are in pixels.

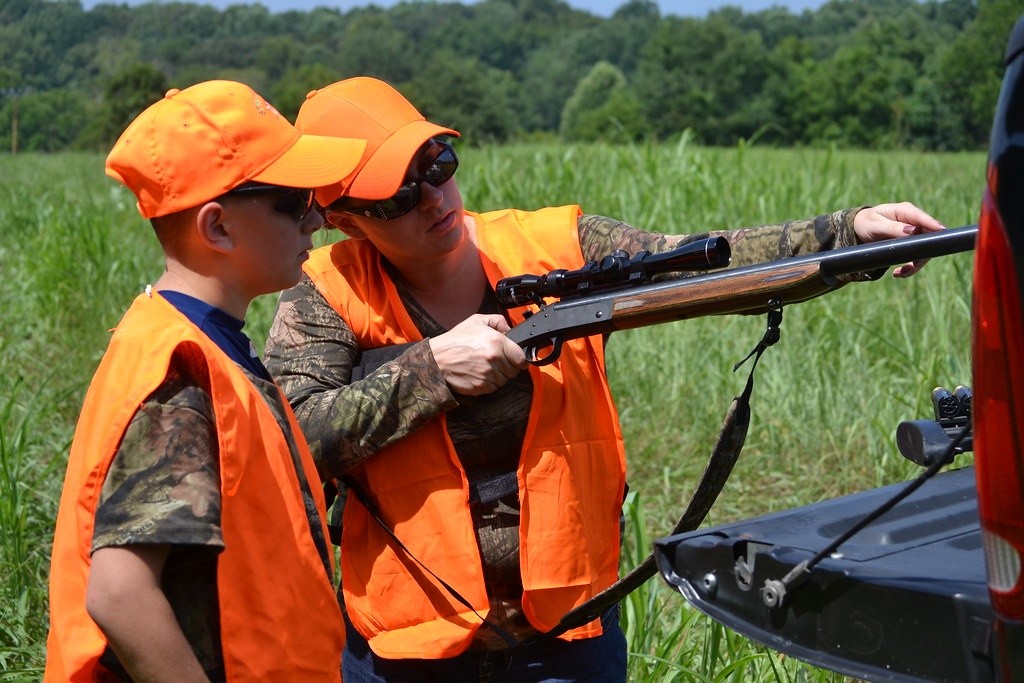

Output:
[105, 81, 370, 220]
[287, 77, 461, 209]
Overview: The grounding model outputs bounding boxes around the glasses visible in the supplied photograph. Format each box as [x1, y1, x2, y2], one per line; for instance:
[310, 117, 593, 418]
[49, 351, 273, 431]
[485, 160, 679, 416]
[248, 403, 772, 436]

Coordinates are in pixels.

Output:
[332, 140, 460, 221]
[234, 182, 316, 221]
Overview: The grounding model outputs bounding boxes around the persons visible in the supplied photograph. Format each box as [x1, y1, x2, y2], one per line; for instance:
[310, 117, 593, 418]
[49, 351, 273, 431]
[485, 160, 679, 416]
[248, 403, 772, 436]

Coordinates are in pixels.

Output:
[41, 79, 369, 683]
[263, 76, 947, 683]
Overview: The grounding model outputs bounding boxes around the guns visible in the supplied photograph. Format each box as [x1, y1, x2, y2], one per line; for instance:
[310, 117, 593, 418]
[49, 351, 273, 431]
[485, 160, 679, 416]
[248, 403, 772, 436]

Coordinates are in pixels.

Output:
[349, 225, 982, 370]
